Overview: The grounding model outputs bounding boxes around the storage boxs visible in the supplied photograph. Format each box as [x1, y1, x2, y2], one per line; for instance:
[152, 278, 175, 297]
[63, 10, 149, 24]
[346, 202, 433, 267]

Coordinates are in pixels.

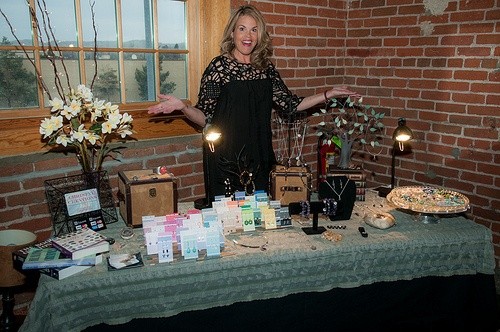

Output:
[117, 168, 178, 228]
[269, 165, 312, 206]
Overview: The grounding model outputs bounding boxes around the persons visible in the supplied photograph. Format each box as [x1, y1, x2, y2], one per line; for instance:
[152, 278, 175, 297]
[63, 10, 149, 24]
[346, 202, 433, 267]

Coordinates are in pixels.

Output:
[147, 5, 361, 208]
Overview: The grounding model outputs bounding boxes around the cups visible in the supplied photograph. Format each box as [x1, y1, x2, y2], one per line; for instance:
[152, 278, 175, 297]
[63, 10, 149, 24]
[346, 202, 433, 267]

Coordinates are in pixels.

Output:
[120, 224, 135, 239]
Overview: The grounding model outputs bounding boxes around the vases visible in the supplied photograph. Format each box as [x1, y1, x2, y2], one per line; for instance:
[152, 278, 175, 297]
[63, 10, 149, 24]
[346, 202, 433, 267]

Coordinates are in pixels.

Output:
[336, 137, 353, 169]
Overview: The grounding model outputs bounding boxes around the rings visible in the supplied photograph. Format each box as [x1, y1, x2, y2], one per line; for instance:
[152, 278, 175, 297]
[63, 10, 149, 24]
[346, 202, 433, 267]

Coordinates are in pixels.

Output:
[348, 95, 350, 97]
[161, 106, 163, 108]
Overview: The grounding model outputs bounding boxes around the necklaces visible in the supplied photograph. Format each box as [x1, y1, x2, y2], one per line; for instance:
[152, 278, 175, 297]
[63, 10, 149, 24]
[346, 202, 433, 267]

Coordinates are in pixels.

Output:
[325, 179, 349, 200]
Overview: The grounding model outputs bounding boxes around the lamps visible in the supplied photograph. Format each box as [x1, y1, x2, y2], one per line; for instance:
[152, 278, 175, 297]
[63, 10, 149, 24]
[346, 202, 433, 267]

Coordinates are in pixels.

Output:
[0, 229, 37, 332]
[387, 116, 414, 190]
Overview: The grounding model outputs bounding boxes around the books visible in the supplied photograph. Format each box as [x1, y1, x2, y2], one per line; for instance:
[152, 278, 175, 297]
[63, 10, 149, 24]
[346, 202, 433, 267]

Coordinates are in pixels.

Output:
[13, 238, 102, 280]
[51, 228, 109, 259]
[22, 248, 96, 269]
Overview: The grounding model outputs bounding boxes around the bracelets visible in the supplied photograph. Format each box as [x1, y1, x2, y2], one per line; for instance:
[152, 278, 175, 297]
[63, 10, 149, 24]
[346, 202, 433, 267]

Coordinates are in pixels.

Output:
[299, 200, 310, 218]
[324, 90, 329, 102]
[322, 198, 337, 216]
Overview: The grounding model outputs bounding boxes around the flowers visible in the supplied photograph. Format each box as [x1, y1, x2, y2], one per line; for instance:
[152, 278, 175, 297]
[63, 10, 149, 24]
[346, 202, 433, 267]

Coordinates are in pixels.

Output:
[311, 95, 383, 144]
[40, 86, 135, 167]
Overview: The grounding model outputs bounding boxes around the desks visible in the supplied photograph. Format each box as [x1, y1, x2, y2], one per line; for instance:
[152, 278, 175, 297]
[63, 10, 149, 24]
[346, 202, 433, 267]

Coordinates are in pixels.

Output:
[16, 190, 498, 332]
